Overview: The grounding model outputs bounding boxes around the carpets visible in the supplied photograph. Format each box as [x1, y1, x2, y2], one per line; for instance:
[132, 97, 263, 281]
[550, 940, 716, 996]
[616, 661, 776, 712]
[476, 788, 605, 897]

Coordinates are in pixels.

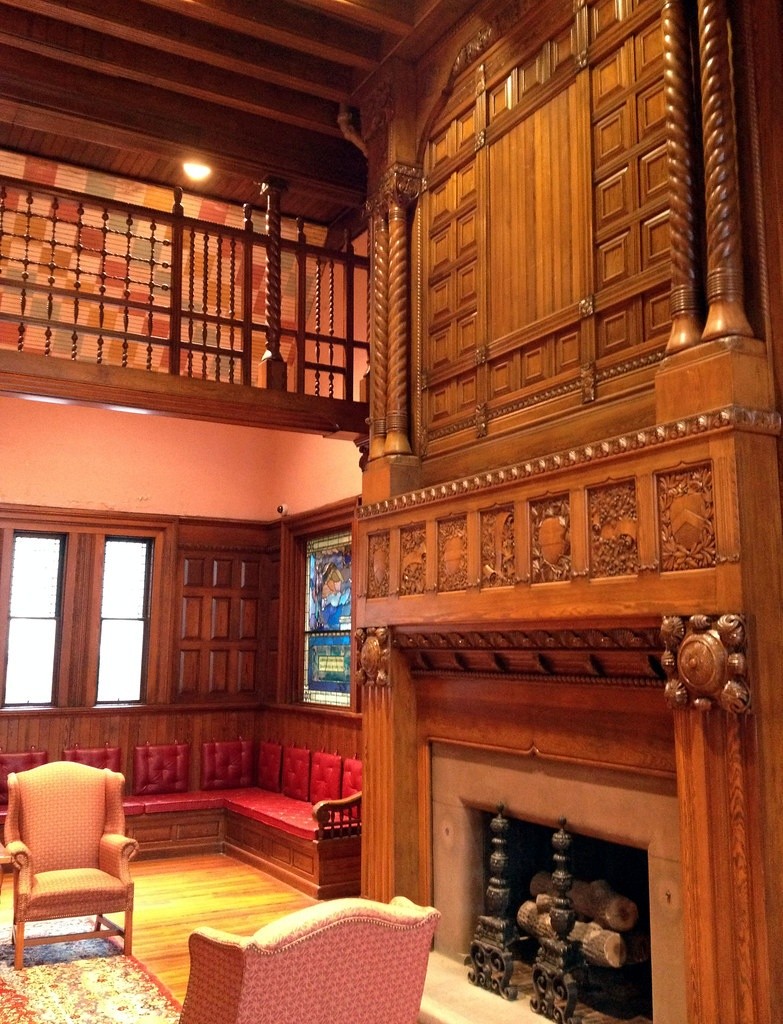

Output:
[0, 915, 182, 1024]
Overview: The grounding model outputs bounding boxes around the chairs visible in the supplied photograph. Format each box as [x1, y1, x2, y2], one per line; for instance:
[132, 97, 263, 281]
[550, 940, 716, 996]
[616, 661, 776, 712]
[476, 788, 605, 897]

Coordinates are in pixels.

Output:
[4, 761, 139, 970]
[179, 896, 441, 1024]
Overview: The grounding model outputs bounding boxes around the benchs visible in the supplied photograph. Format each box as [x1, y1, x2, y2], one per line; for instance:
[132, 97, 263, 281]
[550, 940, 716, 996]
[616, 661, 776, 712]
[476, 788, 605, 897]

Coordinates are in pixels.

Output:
[0, 741, 369, 901]
